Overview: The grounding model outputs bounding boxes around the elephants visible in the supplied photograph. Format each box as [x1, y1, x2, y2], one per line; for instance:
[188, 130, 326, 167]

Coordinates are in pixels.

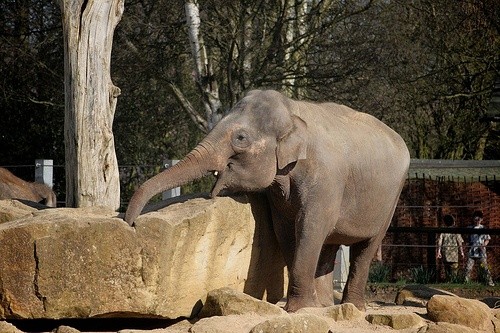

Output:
[124, 88, 410, 314]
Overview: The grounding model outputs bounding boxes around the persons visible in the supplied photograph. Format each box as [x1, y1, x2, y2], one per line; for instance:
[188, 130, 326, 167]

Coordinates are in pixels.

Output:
[436, 214, 465, 283]
[462, 210, 496, 288]
